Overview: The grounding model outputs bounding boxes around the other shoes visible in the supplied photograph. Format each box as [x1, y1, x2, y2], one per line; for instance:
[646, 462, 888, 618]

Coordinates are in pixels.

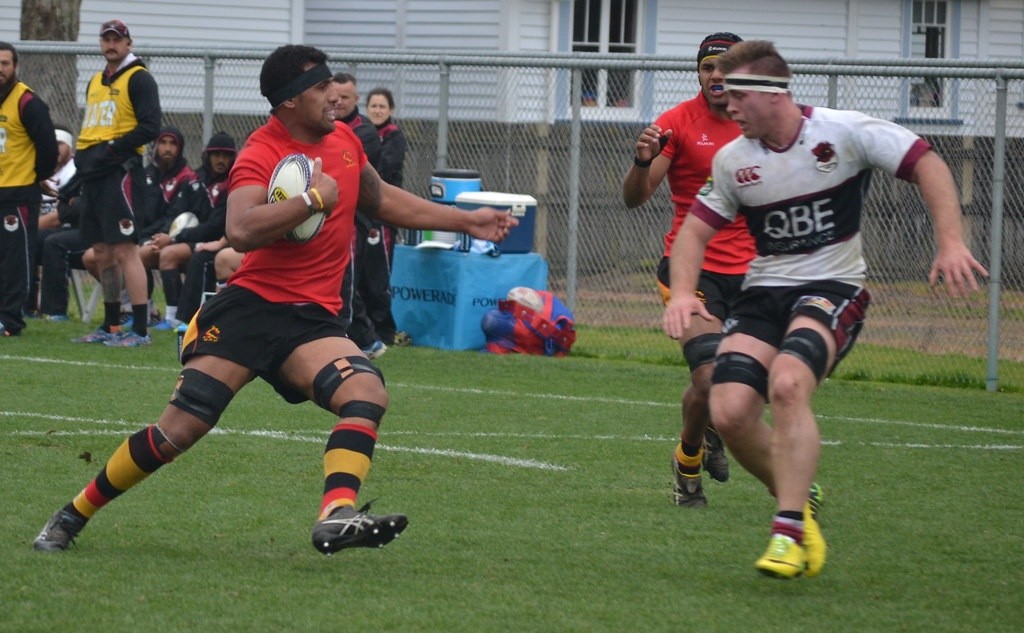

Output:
[45, 313, 70, 322]
[0, 322, 21, 338]
[20, 308, 39, 319]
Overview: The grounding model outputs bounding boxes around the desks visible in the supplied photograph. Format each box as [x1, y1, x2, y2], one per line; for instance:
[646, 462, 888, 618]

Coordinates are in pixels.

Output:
[387, 243, 548, 353]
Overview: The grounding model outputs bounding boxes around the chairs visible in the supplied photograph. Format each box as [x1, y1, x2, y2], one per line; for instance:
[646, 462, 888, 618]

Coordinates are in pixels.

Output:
[68, 250, 103, 322]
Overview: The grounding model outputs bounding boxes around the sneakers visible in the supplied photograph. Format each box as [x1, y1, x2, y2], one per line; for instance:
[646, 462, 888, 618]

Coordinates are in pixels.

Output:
[387, 331, 412, 346]
[312, 496, 408, 557]
[700, 424, 729, 482]
[355, 340, 387, 361]
[103, 329, 151, 348]
[671, 455, 706, 509]
[799, 480, 827, 577]
[119, 313, 188, 333]
[69, 327, 121, 343]
[756, 520, 805, 580]
[33, 509, 86, 553]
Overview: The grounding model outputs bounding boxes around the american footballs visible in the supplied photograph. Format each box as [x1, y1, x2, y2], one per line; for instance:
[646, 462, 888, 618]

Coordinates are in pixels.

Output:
[169, 211, 200, 243]
[507, 286, 544, 314]
[267, 153, 327, 245]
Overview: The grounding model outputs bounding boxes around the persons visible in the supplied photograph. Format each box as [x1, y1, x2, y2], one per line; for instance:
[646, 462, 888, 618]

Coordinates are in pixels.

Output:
[34, 42, 520, 557]
[0, 41, 59, 338]
[332, 71, 387, 360]
[119, 123, 193, 328]
[661, 39, 990, 580]
[622, 32, 757, 506]
[140, 130, 237, 333]
[24, 125, 78, 230]
[70, 20, 161, 348]
[360, 87, 406, 346]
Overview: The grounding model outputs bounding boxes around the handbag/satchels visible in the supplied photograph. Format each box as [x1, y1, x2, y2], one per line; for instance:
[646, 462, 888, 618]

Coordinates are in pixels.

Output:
[480, 286, 577, 357]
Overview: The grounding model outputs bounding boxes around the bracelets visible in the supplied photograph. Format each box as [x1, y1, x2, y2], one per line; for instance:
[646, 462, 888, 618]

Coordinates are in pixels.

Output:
[311, 188, 324, 210]
[302, 192, 315, 215]
[634, 153, 651, 168]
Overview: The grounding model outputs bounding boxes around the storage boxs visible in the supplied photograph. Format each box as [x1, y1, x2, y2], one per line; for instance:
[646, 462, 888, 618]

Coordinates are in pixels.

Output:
[456, 190, 538, 254]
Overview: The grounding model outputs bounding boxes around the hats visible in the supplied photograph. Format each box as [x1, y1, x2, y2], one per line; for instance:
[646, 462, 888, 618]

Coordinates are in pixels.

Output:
[100, 20, 130, 38]
[204, 130, 236, 153]
